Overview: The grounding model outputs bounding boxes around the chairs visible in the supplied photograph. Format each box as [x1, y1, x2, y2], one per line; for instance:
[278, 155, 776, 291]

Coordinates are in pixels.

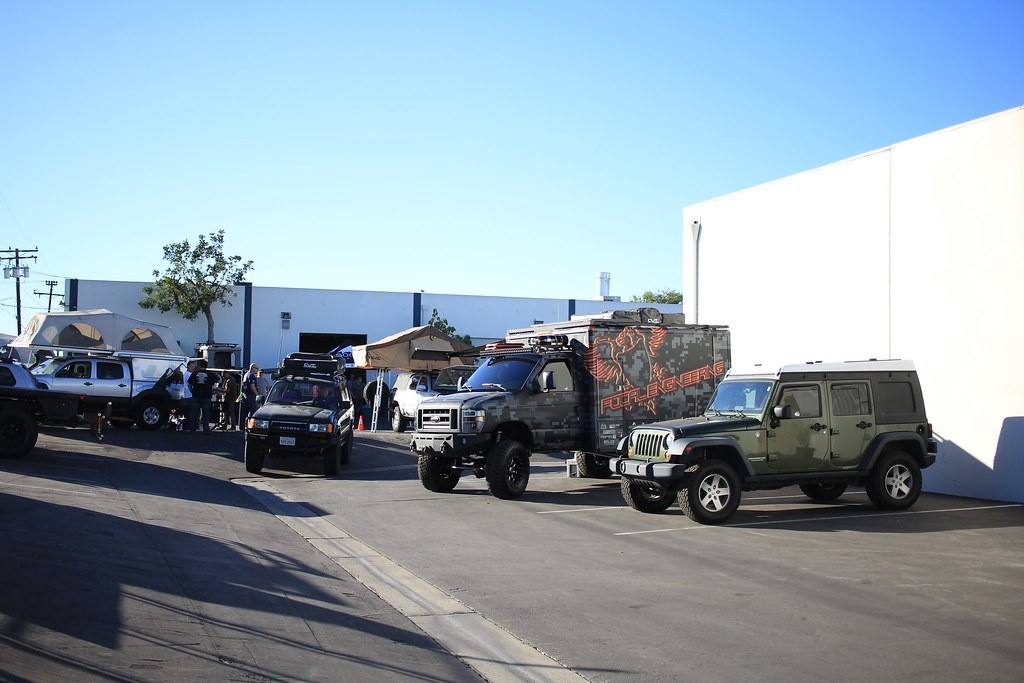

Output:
[278, 391, 296, 404]
[74, 366, 85, 378]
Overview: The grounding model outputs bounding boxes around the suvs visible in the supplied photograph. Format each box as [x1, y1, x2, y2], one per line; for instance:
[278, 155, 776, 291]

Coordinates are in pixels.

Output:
[243, 351, 356, 477]
[608, 357, 939, 525]
[386, 371, 459, 433]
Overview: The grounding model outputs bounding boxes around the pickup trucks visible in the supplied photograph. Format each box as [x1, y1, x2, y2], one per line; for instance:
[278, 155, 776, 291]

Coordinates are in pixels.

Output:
[28, 357, 185, 432]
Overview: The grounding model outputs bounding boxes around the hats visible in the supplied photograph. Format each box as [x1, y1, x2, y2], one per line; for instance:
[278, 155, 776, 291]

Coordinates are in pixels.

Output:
[250, 363, 259, 368]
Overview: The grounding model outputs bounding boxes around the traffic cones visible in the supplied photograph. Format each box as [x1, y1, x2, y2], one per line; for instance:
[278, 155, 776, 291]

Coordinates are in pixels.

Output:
[355, 415, 365, 430]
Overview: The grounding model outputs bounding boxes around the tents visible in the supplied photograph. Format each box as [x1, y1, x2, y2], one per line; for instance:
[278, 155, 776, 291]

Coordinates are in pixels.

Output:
[6, 308, 186, 368]
[350, 324, 481, 433]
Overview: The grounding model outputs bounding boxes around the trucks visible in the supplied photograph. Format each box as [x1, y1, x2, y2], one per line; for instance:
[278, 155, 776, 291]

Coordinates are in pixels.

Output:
[408, 307, 734, 500]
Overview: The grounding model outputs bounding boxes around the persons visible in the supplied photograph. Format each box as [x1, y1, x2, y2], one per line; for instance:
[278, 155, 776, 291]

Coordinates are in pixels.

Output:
[240, 363, 260, 430]
[347, 375, 370, 430]
[312, 385, 337, 409]
[218, 371, 237, 430]
[188, 360, 222, 433]
[256, 369, 269, 406]
[181, 362, 201, 431]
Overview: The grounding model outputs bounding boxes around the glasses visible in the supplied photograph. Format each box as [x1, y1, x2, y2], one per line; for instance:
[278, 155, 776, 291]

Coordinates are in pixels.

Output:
[259, 372, 264, 374]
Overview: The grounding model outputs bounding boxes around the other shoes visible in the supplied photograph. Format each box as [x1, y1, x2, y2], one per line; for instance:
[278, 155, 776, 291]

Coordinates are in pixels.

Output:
[228, 427, 236, 430]
[218, 427, 226, 430]
[190, 431, 196, 434]
[203, 431, 212, 435]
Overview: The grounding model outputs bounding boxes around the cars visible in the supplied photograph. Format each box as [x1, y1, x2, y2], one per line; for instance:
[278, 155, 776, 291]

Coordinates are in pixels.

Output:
[1, 361, 39, 390]
